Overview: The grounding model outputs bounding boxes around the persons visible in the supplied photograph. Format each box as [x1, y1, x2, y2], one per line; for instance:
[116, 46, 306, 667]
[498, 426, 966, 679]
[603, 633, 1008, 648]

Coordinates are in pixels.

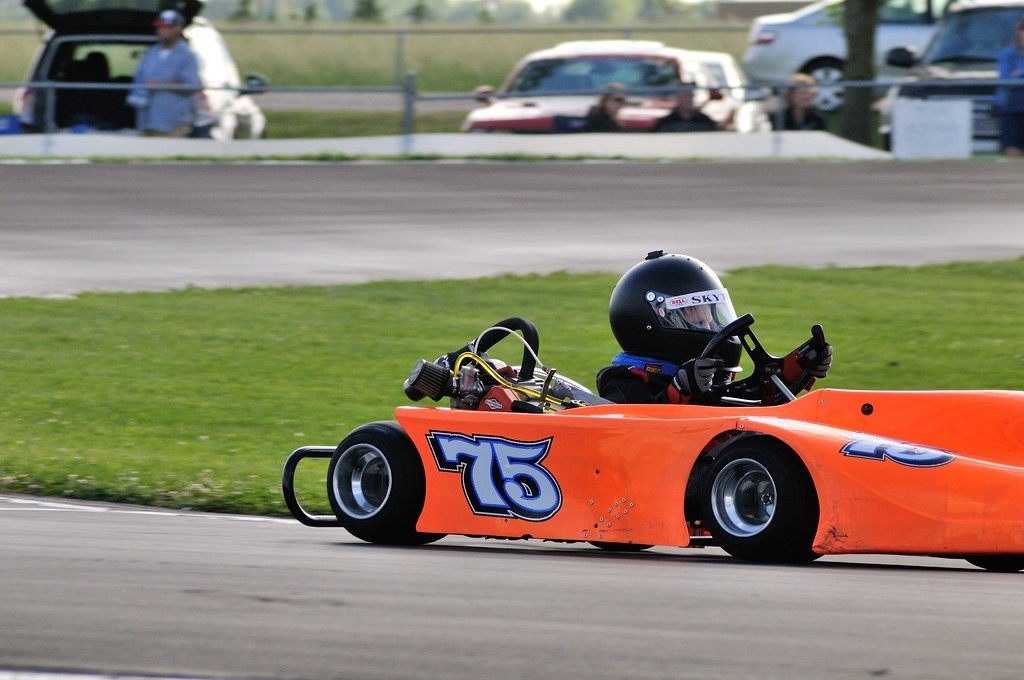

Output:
[582, 83, 625, 133]
[650, 91, 719, 132]
[764, 74, 827, 131]
[595, 250, 834, 447]
[993, 16, 1024, 156]
[126, 10, 215, 137]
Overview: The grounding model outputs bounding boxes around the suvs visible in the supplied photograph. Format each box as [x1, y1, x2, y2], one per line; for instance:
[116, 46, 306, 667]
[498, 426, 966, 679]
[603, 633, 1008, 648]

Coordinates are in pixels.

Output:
[870, 1, 1023, 157]
[11, 1, 271, 139]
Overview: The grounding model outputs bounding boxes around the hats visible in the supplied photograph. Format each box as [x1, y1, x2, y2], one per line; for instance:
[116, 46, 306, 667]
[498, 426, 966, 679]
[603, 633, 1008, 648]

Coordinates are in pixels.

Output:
[155, 10, 185, 27]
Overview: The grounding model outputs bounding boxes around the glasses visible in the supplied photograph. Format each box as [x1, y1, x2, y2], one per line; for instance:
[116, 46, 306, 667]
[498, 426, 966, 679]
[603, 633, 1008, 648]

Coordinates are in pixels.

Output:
[609, 96, 624, 102]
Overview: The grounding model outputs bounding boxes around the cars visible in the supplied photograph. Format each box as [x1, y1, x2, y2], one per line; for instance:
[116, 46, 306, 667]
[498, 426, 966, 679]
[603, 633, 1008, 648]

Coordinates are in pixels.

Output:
[746, 0, 954, 112]
[458, 40, 737, 132]
[579, 52, 773, 133]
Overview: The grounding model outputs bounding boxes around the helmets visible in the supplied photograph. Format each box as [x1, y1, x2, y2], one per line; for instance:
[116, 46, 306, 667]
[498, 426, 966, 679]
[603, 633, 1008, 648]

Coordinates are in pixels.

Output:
[609, 250, 743, 372]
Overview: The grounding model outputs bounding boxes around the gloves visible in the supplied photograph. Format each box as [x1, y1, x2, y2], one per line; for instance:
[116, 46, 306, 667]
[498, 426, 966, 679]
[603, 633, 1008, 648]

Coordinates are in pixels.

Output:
[667, 358, 723, 404]
[782, 341, 832, 383]
